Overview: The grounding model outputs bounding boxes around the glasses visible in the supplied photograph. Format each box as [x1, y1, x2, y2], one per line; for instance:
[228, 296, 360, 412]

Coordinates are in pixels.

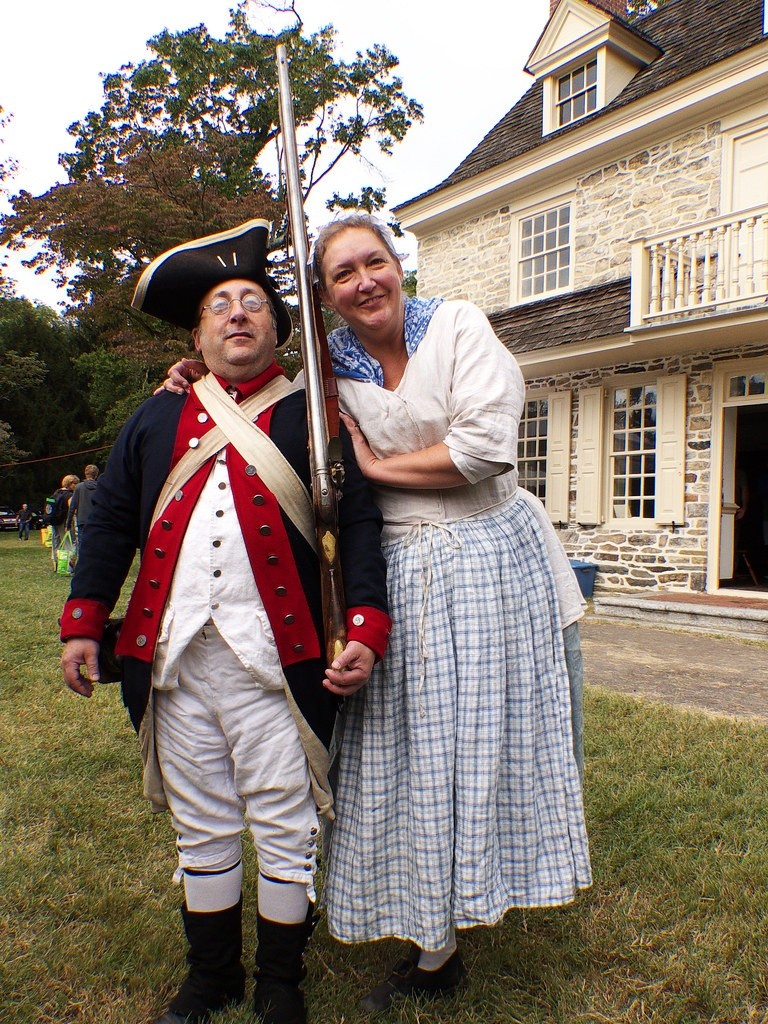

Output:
[199, 293, 278, 323]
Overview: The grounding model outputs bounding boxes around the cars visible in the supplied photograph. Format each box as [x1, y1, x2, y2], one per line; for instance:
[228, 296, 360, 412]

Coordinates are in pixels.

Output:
[0, 506, 45, 531]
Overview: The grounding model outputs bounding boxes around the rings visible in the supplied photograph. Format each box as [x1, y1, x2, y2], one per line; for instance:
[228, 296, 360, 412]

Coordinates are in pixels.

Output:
[354, 424, 360, 427]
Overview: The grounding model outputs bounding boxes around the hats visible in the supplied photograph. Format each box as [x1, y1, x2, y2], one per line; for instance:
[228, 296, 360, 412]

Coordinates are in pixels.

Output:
[130, 218, 293, 350]
[306, 208, 419, 285]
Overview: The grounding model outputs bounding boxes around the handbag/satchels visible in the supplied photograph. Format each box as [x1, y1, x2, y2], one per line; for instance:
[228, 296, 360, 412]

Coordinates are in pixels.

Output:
[54, 530, 79, 577]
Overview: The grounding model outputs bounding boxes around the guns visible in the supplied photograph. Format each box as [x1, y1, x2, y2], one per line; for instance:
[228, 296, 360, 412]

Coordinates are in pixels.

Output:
[274, 40, 353, 677]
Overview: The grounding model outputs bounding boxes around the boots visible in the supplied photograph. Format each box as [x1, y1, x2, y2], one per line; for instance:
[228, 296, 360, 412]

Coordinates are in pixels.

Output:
[252, 903, 321, 1024]
[159, 890, 246, 1024]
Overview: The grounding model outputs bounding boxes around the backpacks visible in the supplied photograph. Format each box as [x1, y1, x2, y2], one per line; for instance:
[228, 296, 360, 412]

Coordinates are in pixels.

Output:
[43, 489, 71, 526]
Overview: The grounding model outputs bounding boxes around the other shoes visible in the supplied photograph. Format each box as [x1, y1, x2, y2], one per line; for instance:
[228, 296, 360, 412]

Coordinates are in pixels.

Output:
[356, 944, 418, 1015]
[409, 948, 463, 1004]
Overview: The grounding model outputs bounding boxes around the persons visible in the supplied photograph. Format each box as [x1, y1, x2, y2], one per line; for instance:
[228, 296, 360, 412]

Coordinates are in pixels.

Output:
[152, 184, 593, 1003]
[15, 464, 98, 574]
[60, 217, 390, 1024]
